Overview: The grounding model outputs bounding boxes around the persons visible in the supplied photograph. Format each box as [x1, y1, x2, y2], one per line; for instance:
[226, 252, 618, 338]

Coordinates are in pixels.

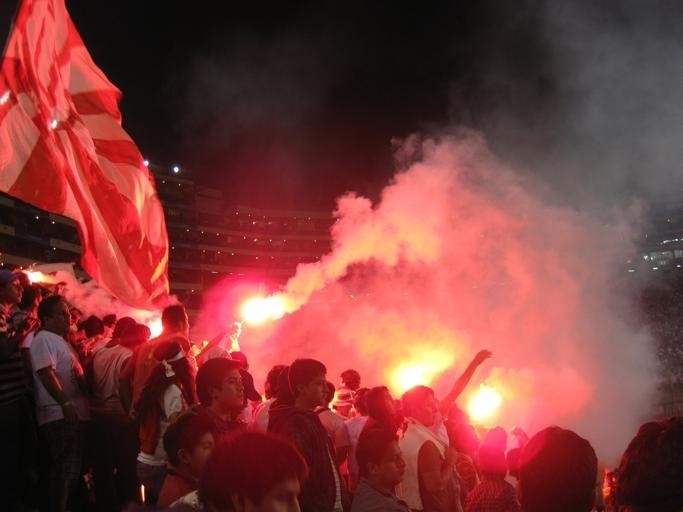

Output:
[506, 427, 530, 504]
[328, 370, 361, 491]
[30, 295, 93, 490]
[198, 431, 309, 512]
[313, 382, 344, 441]
[104, 314, 118, 338]
[602, 416, 682, 512]
[344, 389, 369, 511]
[264, 364, 292, 400]
[484, 427, 508, 454]
[135, 341, 199, 511]
[443, 408, 467, 437]
[130, 305, 198, 420]
[156, 413, 215, 511]
[79, 315, 112, 377]
[91, 317, 138, 462]
[453, 425, 480, 502]
[360, 385, 396, 432]
[3, 263, 31, 287]
[1, 269, 42, 490]
[45, 282, 83, 351]
[398, 386, 462, 511]
[181, 357, 245, 445]
[195, 324, 237, 359]
[463, 442, 521, 512]
[17, 285, 44, 370]
[202, 345, 233, 364]
[138, 323, 151, 346]
[231, 352, 263, 409]
[349, 428, 420, 512]
[268, 358, 348, 511]
[513, 426, 598, 511]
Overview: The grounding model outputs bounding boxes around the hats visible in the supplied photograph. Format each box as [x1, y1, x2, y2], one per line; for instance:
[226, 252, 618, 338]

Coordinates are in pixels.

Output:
[0, 269, 22, 285]
